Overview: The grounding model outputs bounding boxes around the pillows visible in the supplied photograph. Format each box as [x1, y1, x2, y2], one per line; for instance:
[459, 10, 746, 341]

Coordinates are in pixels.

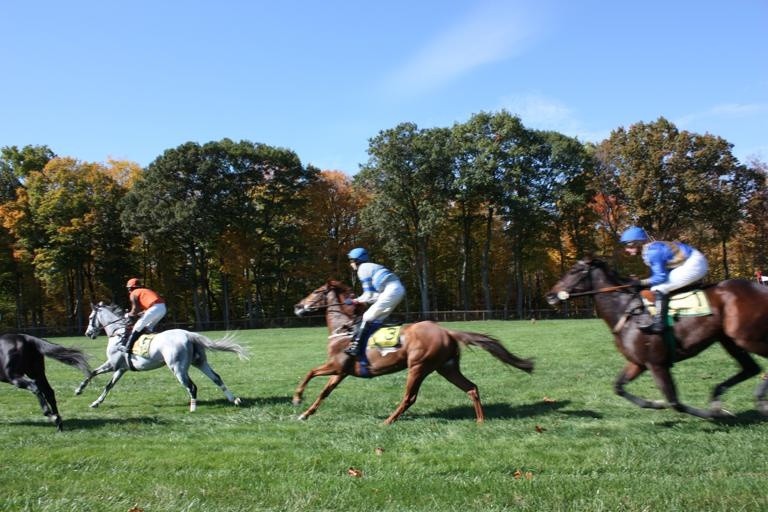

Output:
[618, 227, 648, 242]
[126, 278, 140, 288]
[348, 248, 368, 261]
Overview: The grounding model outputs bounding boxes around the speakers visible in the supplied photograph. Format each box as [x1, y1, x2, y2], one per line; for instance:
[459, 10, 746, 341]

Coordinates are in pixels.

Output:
[344, 321, 371, 356]
[643, 289, 668, 333]
[121, 330, 138, 352]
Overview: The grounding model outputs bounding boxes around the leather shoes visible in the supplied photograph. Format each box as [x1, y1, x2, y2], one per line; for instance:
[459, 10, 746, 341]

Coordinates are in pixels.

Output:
[345, 298, 358, 305]
[630, 279, 640, 286]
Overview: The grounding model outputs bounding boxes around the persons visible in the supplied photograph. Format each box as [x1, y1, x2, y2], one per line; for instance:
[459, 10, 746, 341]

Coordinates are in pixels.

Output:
[616, 224, 710, 336]
[113, 277, 168, 354]
[339, 246, 407, 362]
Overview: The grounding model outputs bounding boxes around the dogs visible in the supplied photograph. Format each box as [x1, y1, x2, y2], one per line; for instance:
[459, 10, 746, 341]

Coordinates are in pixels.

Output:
[0, 331, 98, 430]
[289, 274, 535, 428]
[71, 299, 252, 413]
[542, 251, 768, 424]
